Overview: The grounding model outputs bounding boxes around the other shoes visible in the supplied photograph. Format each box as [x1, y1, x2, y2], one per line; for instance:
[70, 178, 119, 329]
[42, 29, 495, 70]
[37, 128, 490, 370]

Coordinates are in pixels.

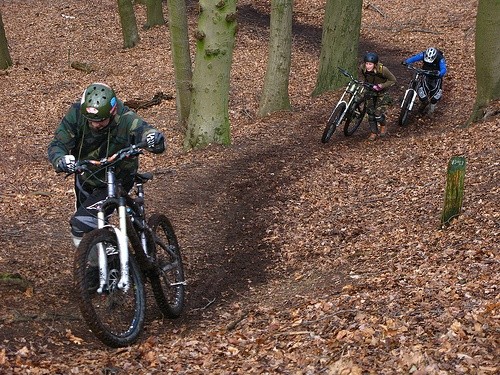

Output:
[379, 123, 387, 135]
[418, 100, 429, 111]
[368, 132, 377, 141]
[83, 267, 99, 291]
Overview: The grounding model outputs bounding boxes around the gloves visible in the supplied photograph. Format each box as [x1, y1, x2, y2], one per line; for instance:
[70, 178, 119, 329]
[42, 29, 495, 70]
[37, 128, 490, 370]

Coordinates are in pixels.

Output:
[56, 154, 76, 174]
[144, 132, 165, 154]
[434, 70, 440, 77]
[401, 61, 407, 68]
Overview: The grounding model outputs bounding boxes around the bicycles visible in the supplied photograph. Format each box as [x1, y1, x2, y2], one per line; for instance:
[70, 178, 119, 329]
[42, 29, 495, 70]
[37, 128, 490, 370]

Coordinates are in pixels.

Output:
[397, 62, 444, 126]
[320, 67, 377, 142]
[58, 136, 186, 344]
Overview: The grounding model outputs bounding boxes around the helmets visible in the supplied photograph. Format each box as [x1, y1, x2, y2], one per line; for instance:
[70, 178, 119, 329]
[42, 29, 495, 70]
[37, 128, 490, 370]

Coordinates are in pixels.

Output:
[81, 82, 117, 121]
[364, 52, 378, 66]
[423, 47, 437, 63]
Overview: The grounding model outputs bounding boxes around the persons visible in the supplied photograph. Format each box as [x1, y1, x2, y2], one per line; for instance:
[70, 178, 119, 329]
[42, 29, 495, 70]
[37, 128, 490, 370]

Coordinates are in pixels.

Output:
[359, 53, 396, 141]
[402, 47, 447, 113]
[47, 83, 165, 294]
